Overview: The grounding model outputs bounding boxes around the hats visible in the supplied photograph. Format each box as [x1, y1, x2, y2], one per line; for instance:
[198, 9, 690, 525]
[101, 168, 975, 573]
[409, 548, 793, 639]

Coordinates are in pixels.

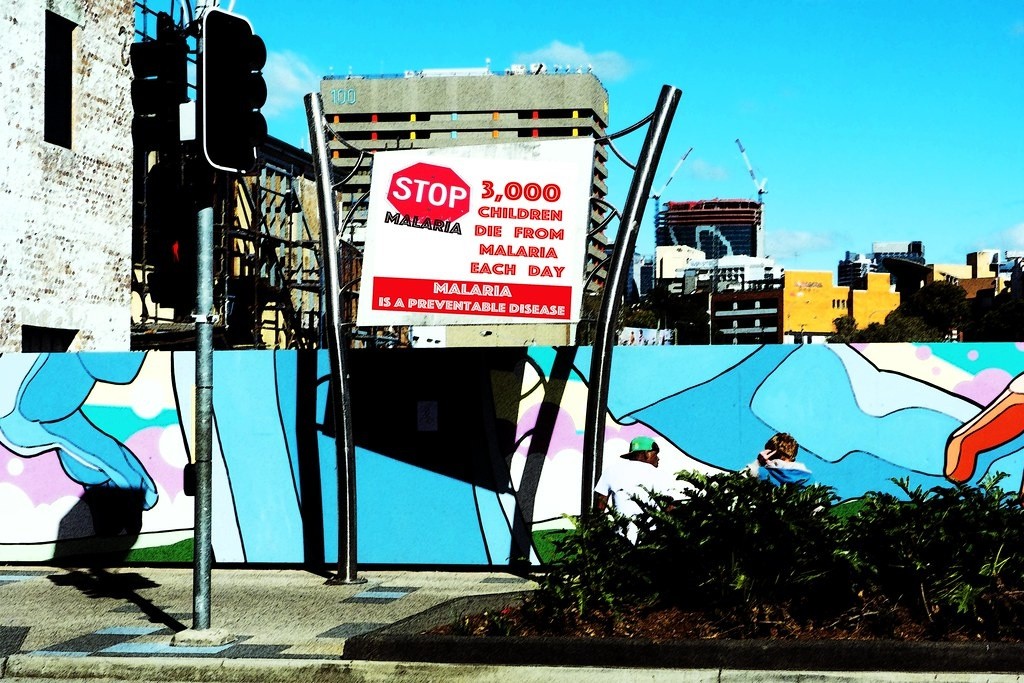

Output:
[620, 435, 656, 459]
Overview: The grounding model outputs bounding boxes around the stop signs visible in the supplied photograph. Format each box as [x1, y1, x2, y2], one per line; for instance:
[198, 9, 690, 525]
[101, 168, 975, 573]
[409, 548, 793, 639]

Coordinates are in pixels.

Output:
[384, 162, 473, 228]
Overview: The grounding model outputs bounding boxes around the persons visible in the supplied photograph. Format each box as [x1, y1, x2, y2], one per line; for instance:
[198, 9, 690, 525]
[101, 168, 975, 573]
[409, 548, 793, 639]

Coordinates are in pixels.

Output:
[755, 432, 814, 492]
[594, 437, 675, 545]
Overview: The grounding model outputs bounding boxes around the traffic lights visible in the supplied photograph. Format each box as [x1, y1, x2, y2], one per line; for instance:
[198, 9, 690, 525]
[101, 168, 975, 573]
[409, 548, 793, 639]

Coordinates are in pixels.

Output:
[202, 6, 269, 171]
[129, 14, 190, 161]
[146, 169, 190, 308]
